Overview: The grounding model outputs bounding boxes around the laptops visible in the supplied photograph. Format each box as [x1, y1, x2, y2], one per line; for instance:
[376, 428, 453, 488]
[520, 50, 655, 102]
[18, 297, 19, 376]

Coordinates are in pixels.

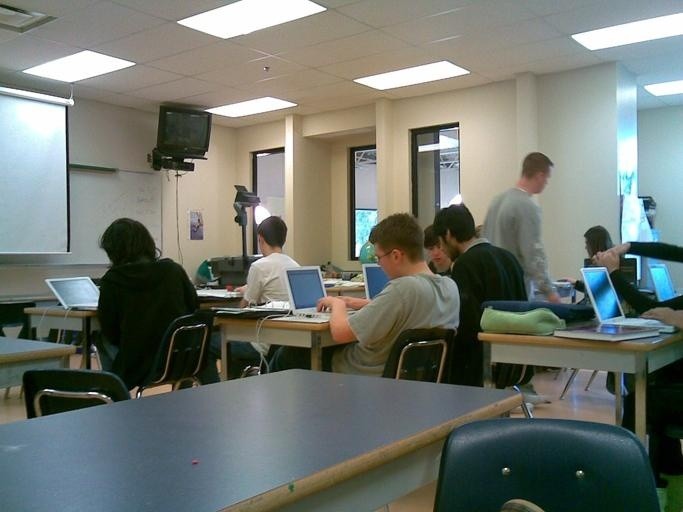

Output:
[44, 276, 100, 311]
[362, 263, 389, 299]
[283, 265, 360, 320]
[580, 266, 681, 333]
[647, 264, 676, 302]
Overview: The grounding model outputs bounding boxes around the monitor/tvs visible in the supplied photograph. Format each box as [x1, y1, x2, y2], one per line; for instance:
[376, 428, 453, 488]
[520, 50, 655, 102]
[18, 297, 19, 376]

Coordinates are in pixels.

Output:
[158, 105, 212, 160]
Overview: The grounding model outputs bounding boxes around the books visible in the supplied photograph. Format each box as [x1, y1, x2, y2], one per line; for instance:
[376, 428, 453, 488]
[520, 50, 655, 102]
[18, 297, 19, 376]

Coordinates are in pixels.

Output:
[210, 300, 290, 315]
[552, 323, 661, 342]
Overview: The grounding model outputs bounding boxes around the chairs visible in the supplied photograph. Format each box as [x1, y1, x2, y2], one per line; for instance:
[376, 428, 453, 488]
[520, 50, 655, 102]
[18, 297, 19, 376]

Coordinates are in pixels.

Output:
[377, 280, 602, 422]
[22, 369, 130, 421]
[433, 417, 660, 512]
[2, 301, 303, 410]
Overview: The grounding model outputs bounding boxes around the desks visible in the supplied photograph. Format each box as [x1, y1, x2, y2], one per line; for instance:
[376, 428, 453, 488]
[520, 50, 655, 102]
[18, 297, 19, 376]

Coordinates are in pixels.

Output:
[1, 282, 365, 388]
[0, 367, 524, 511]
[476, 322, 683, 448]
[209, 307, 363, 381]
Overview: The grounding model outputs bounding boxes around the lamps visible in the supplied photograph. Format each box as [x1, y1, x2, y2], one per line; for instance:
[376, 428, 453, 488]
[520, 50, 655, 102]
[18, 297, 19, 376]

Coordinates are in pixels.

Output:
[231, 183, 270, 257]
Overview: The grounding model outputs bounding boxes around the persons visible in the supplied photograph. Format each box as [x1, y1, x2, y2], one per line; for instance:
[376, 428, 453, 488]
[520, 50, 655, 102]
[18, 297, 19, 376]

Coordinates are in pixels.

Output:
[422, 223, 453, 274]
[561, 225, 616, 306]
[589, 239, 683, 322]
[262, 210, 462, 379]
[431, 203, 534, 388]
[95, 216, 201, 393]
[618, 305, 682, 487]
[197, 215, 305, 384]
[477, 151, 563, 307]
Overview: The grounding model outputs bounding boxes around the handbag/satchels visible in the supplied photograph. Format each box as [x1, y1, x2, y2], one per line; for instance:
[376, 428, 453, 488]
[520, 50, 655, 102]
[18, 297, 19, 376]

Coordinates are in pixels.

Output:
[481, 305, 565, 335]
